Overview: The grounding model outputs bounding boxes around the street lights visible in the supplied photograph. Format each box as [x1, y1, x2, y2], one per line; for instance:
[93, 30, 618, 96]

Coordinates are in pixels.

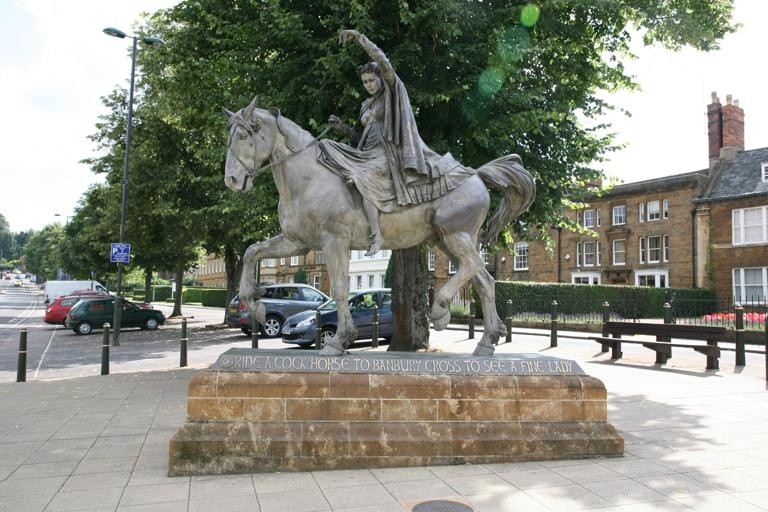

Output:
[100, 27, 172, 347]
[52, 209, 76, 229]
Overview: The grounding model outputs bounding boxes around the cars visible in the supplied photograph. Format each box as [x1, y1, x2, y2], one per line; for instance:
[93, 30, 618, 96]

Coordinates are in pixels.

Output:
[224, 280, 392, 346]
[0, 268, 37, 288]
[40, 278, 166, 338]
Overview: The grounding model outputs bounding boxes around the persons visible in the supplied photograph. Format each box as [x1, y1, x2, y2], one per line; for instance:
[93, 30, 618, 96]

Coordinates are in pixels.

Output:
[317, 28, 478, 258]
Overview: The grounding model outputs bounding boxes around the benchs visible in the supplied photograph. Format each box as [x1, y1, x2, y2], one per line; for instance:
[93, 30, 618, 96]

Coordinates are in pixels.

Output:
[596, 321, 726, 369]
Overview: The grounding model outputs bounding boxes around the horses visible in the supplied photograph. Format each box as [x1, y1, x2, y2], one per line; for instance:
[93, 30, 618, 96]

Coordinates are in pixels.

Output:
[220, 94, 538, 357]
